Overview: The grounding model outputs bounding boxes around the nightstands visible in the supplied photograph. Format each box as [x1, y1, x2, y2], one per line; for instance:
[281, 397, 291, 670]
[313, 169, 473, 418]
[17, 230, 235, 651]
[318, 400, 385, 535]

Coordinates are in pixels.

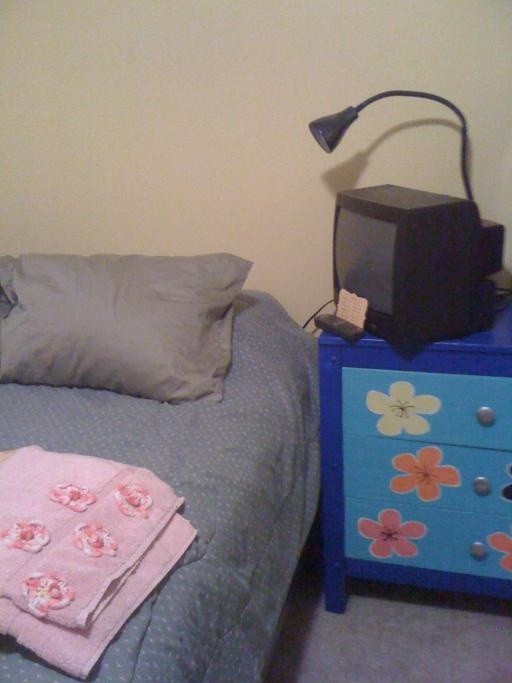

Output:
[318, 293, 511, 614]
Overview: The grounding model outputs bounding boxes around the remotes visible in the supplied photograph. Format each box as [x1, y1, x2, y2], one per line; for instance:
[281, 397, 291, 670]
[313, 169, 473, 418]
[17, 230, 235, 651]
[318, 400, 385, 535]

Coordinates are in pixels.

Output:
[314, 314, 365, 342]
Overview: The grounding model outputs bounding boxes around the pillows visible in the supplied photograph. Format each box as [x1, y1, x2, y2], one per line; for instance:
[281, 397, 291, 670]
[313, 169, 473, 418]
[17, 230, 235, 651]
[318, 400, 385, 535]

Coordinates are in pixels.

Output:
[0, 253, 251, 401]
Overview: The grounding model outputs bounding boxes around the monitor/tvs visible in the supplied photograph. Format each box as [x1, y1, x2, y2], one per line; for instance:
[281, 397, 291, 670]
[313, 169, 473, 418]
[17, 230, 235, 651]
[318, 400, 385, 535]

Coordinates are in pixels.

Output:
[332, 184, 505, 340]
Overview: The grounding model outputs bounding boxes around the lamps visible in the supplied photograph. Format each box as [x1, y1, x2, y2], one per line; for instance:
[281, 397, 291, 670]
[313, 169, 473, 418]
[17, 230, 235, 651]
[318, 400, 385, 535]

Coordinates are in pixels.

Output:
[309, 92, 512, 312]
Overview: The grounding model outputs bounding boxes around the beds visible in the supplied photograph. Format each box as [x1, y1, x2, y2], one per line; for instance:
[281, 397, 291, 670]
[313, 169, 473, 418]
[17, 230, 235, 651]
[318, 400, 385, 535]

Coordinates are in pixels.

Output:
[1, 290, 321, 681]
